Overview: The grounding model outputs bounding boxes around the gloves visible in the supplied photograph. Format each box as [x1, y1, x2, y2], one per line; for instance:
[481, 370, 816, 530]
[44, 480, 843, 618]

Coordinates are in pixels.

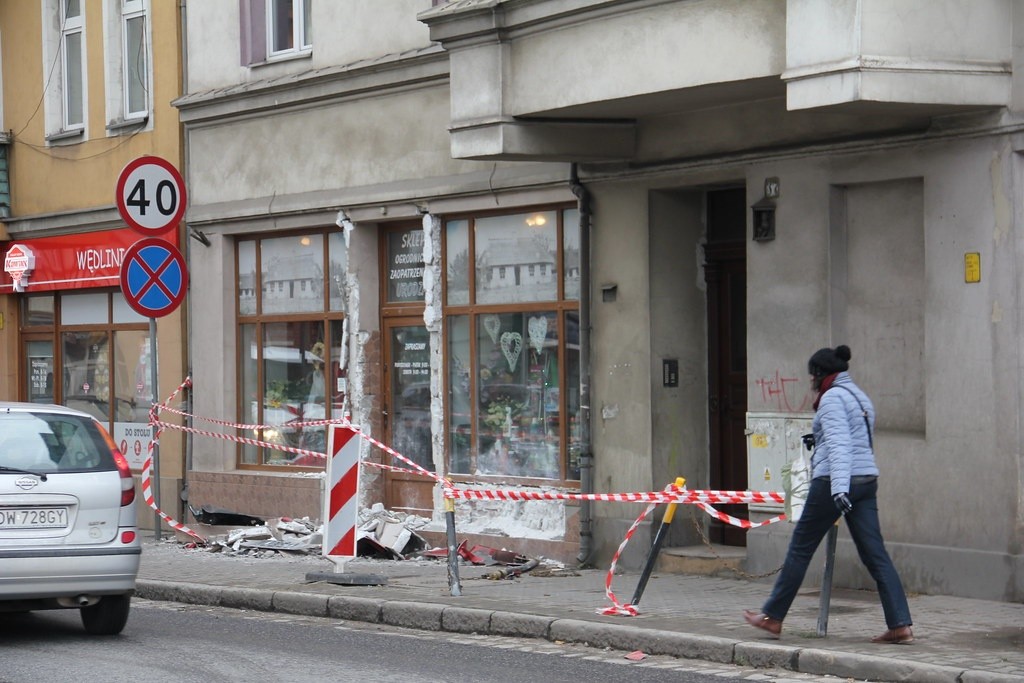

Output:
[802, 434, 816, 452]
[834, 492, 854, 516]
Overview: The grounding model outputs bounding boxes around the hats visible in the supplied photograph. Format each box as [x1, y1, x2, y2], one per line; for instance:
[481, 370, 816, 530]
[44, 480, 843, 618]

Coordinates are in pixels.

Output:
[811, 345, 851, 373]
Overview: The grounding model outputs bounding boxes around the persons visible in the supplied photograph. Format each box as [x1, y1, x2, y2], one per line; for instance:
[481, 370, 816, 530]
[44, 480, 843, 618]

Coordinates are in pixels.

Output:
[742, 346, 916, 644]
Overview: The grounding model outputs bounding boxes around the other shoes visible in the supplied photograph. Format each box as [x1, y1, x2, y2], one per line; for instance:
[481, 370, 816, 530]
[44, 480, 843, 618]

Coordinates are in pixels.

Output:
[872, 625, 915, 644]
[744, 609, 782, 638]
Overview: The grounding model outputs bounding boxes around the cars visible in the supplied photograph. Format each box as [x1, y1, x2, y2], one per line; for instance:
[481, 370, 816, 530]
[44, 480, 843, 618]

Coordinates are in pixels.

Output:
[396, 380, 580, 475]
[0, 401, 142, 635]
[249, 345, 345, 460]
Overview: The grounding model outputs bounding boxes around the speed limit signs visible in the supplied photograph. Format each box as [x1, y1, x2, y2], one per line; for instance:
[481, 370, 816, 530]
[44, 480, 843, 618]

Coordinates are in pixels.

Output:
[116, 156, 187, 235]
